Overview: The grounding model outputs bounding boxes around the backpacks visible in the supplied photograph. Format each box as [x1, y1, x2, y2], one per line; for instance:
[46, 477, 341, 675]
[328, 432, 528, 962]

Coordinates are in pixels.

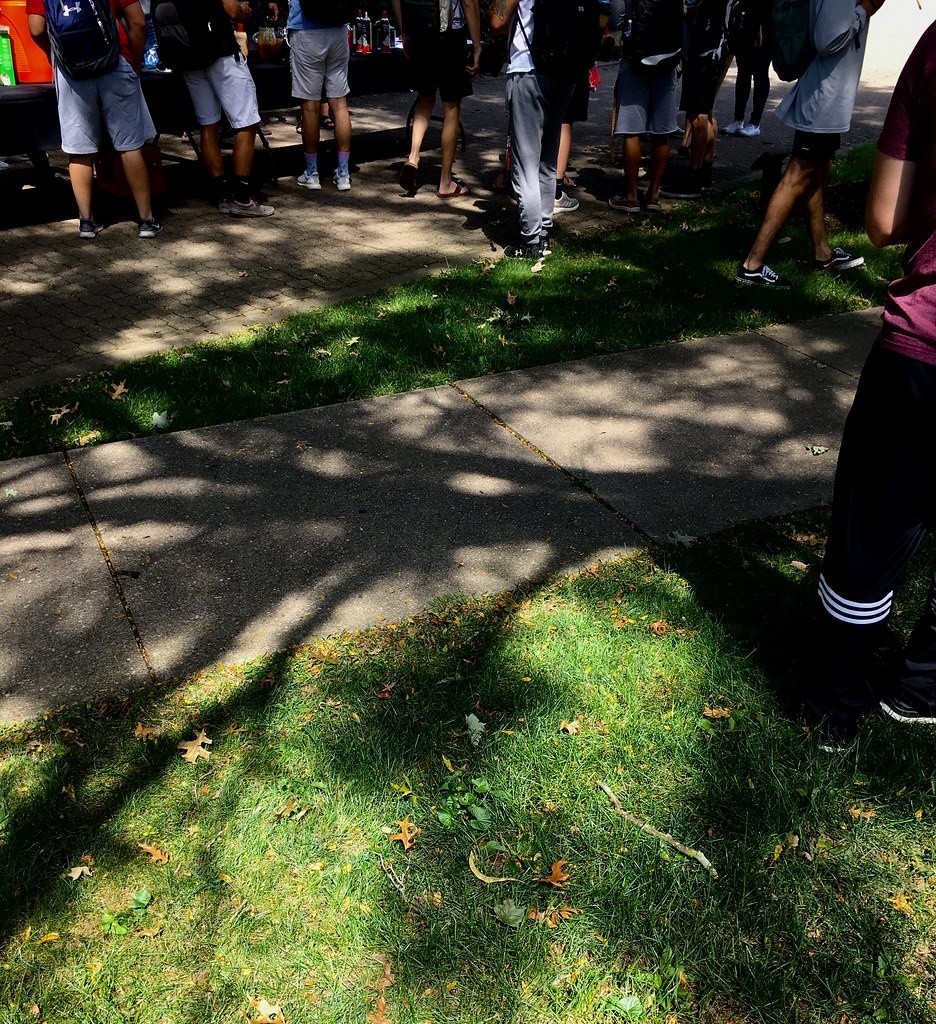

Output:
[150, 0, 240, 71]
[300, 0, 359, 28]
[400, 0, 459, 39]
[514, 0, 601, 83]
[772, 0, 816, 82]
[623, 0, 684, 76]
[44, 0, 120, 82]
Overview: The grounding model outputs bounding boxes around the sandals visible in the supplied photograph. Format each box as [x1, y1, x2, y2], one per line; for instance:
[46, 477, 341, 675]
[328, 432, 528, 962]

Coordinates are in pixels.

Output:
[319, 116, 335, 130]
[297, 120, 302, 133]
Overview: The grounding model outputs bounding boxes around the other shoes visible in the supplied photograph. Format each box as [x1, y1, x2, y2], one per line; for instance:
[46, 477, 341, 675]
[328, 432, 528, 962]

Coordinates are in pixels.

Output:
[668, 144, 690, 165]
[504, 231, 545, 262]
[641, 191, 661, 212]
[609, 197, 640, 213]
[879, 682, 936, 725]
[818, 708, 862, 753]
[539, 233, 552, 254]
[260, 120, 272, 135]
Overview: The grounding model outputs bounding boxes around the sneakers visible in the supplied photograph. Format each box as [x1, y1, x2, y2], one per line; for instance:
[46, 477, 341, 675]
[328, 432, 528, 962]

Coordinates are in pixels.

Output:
[218, 198, 275, 217]
[553, 191, 580, 215]
[740, 123, 761, 137]
[722, 120, 744, 134]
[735, 264, 791, 291]
[79, 218, 104, 238]
[814, 247, 865, 271]
[332, 168, 351, 191]
[296, 171, 321, 190]
[139, 217, 164, 238]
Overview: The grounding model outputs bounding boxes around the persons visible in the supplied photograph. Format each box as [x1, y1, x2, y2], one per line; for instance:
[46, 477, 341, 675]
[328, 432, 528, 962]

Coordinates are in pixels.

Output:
[810, 18, 936, 724]
[0, 0, 885, 289]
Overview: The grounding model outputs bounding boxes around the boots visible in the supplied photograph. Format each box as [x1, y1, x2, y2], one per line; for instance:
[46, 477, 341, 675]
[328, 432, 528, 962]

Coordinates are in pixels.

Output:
[700, 163, 713, 192]
[659, 166, 702, 199]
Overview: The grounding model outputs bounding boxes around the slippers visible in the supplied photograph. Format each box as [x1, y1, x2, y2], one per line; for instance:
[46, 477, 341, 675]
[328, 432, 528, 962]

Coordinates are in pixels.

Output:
[399, 163, 416, 191]
[437, 181, 472, 198]
